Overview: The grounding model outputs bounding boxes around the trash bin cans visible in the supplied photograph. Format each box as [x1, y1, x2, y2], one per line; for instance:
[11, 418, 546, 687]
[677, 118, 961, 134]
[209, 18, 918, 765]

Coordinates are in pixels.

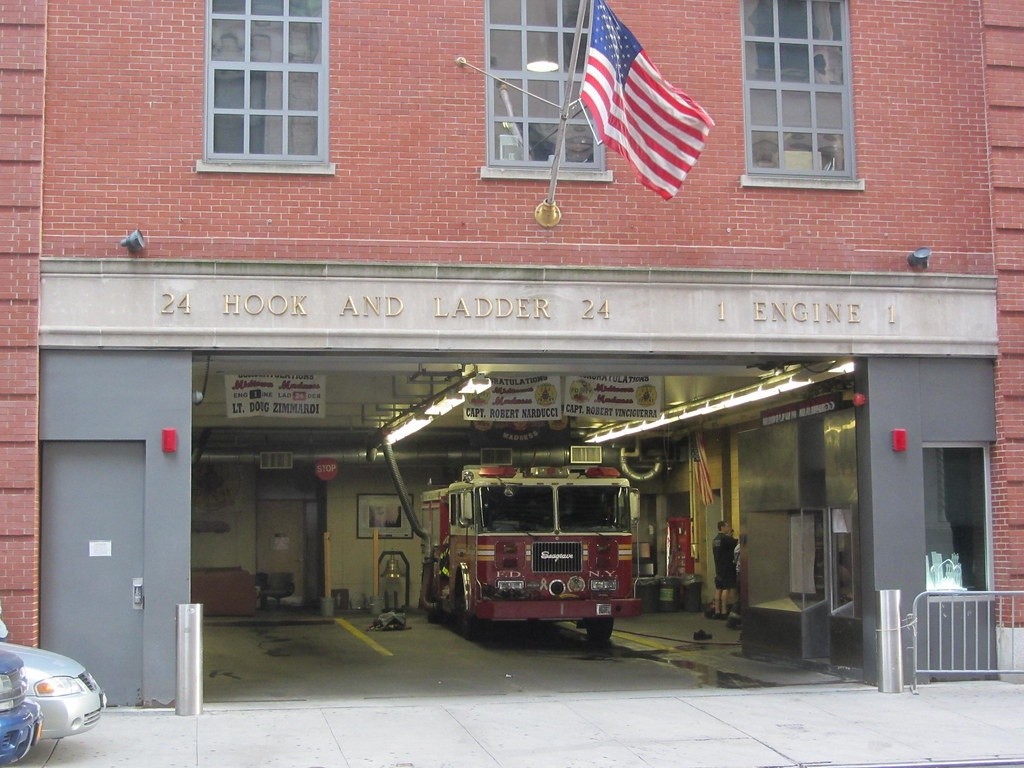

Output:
[683, 574, 701, 613]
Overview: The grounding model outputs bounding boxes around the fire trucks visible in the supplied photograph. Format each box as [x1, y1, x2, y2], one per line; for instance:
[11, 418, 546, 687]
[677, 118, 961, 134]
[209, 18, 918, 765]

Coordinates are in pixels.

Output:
[419, 463, 643, 644]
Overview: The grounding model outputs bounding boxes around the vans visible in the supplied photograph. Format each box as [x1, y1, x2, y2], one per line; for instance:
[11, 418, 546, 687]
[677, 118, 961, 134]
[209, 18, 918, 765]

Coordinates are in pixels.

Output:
[0, 608, 45, 766]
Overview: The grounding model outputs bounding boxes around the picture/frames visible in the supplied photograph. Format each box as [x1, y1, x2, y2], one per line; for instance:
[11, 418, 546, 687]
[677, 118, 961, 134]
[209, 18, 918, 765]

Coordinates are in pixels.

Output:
[357, 493, 414, 539]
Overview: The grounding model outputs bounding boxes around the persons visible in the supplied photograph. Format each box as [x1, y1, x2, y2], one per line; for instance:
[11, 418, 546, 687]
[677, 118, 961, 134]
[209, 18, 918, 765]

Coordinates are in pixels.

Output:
[712, 521, 741, 620]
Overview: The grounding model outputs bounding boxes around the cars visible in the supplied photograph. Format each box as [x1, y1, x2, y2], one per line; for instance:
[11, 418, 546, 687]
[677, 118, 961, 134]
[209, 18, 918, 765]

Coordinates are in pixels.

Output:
[1, 641, 107, 740]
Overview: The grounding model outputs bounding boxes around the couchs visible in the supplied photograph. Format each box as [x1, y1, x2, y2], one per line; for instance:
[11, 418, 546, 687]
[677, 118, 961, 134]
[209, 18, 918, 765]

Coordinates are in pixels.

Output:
[191, 574, 258, 615]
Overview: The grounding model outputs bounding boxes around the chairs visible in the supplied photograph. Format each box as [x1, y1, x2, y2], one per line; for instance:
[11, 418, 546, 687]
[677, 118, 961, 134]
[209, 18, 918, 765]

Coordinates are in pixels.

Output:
[260, 572, 296, 612]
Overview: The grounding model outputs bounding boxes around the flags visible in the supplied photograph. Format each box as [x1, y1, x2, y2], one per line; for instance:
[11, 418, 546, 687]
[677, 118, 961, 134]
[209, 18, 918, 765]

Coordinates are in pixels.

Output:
[580, 0, 715, 201]
[691, 430, 713, 505]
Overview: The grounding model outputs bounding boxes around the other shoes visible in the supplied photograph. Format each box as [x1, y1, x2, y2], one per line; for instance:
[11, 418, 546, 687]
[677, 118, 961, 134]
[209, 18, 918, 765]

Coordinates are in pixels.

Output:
[712, 613, 727, 619]
[693, 630, 712, 640]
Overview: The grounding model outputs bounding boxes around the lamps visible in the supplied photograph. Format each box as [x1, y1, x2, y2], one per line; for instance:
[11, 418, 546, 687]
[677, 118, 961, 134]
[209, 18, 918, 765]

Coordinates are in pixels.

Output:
[582, 358, 855, 443]
[378, 372, 492, 445]
